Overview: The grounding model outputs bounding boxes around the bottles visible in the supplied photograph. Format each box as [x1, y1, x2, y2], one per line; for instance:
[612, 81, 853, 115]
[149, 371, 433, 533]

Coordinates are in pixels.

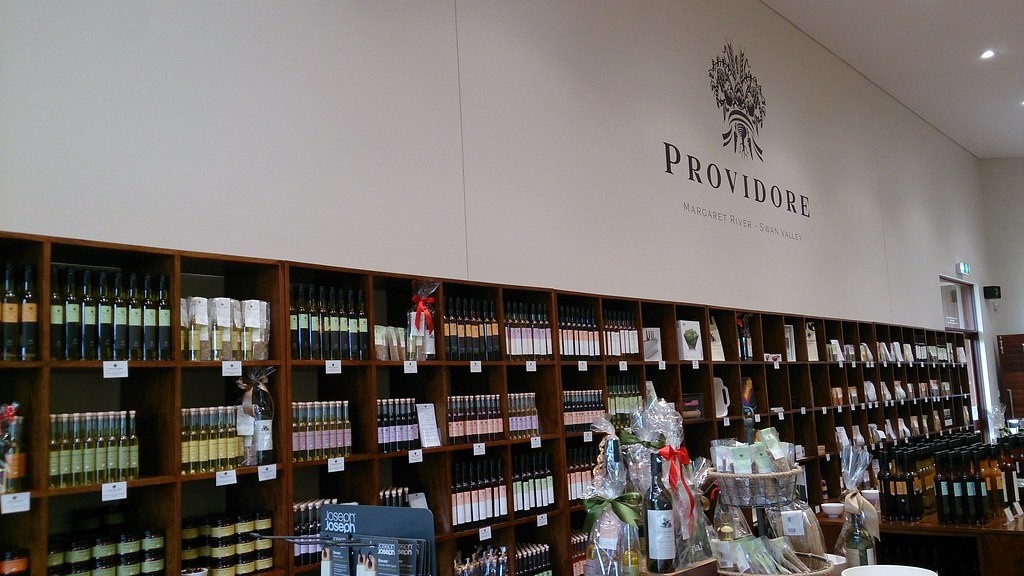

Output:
[586, 531, 598, 576]
[114, 273, 128, 361]
[568, 446, 597, 506]
[6, 416, 26, 492]
[645, 454, 676, 572]
[21, 267, 39, 361]
[98, 271, 113, 361]
[508, 393, 539, 440]
[737, 319, 753, 361]
[717, 506, 734, 571]
[159, 277, 171, 362]
[292, 401, 351, 463]
[0, 265, 21, 360]
[878, 428, 1024, 525]
[143, 276, 158, 361]
[623, 539, 640, 576]
[0, 413, 6, 493]
[570, 529, 588, 576]
[380, 487, 409, 507]
[563, 390, 605, 433]
[505, 302, 552, 361]
[65, 269, 81, 360]
[607, 374, 643, 427]
[846, 510, 874, 567]
[289, 283, 369, 361]
[82, 269, 97, 360]
[377, 398, 419, 452]
[444, 296, 499, 361]
[513, 453, 555, 519]
[407, 304, 434, 360]
[129, 272, 143, 361]
[515, 542, 552, 576]
[560, 306, 601, 361]
[453, 460, 508, 533]
[50, 267, 64, 360]
[293, 498, 337, 567]
[448, 395, 504, 445]
[453, 548, 507, 576]
[181, 315, 268, 361]
[603, 309, 639, 361]
[181, 390, 276, 476]
[50, 411, 138, 488]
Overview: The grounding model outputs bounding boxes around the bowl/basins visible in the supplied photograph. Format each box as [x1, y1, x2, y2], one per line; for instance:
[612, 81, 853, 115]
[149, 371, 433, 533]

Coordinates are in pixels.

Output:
[861, 490, 879, 502]
[821, 503, 844, 517]
[824, 554, 847, 576]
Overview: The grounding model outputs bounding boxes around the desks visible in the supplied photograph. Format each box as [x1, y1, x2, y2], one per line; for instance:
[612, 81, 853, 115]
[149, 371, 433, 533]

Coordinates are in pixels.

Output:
[813, 504, 1024, 576]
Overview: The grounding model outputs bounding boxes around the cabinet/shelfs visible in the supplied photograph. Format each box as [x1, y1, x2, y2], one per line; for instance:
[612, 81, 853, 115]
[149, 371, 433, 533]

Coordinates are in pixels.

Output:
[555, 289, 717, 576]
[0, 230, 290, 576]
[286, 260, 571, 576]
[874, 323, 975, 446]
[707, 306, 885, 536]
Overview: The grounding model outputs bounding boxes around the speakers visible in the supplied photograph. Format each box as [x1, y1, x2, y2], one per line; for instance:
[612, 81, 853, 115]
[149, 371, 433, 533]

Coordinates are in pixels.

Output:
[984, 286, 1001, 299]
